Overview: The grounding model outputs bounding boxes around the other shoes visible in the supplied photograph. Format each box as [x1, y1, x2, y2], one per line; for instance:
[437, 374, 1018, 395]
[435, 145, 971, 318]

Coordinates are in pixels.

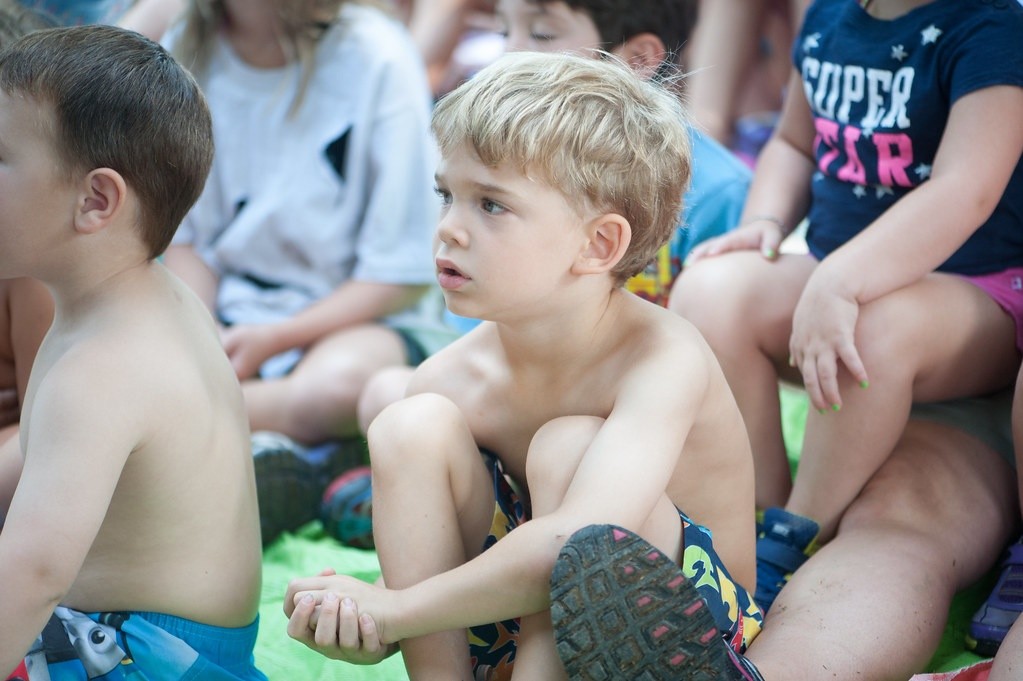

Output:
[321, 467, 371, 550]
[549, 523, 749, 681]
[251, 432, 352, 548]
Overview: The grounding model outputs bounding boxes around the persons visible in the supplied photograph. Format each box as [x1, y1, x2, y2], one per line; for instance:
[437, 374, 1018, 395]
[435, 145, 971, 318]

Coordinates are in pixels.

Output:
[663, 0, 1023, 611]
[1, 1, 812, 539]
[282, 50, 769, 681]
[551, 362, 1023, 680]
[0, 24, 268, 680]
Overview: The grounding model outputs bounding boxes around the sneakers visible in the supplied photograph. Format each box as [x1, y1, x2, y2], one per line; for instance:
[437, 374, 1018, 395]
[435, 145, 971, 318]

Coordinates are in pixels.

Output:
[965, 537, 1023, 657]
[751, 508, 822, 613]
